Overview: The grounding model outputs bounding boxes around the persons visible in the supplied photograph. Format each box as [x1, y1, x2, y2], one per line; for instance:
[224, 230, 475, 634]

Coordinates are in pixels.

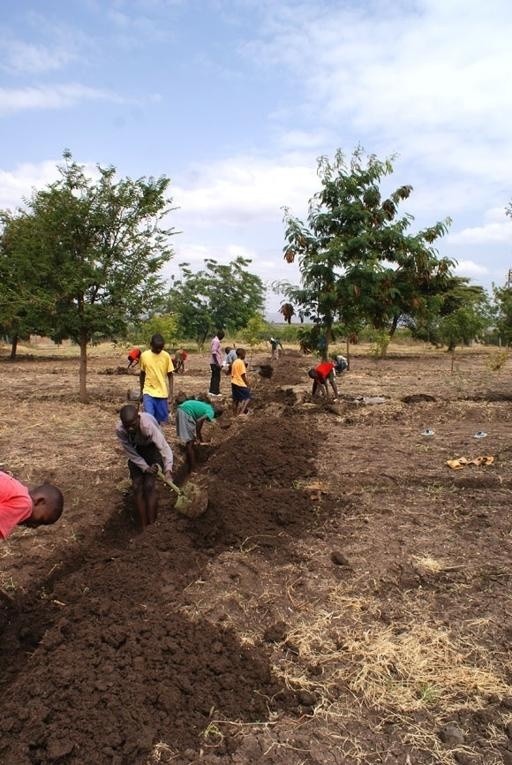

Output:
[0, 472, 63, 540]
[175, 399, 223, 465]
[270, 337, 283, 362]
[127, 348, 142, 368]
[138, 333, 174, 425]
[170, 349, 185, 373]
[224, 347, 248, 375]
[333, 354, 348, 374]
[230, 348, 251, 416]
[308, 363, 339, 398]
[207, 330, 226, 397]
[114, 404, 174, 527]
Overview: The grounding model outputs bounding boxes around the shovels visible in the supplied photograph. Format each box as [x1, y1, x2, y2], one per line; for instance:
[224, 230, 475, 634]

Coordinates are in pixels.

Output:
[151, 461, 209, 520]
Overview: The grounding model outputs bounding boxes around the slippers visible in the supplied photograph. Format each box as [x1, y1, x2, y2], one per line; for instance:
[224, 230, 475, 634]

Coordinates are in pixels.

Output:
[446, 455, 494, 469]
[473, 431, 488, 438]
[420, 430, 435, 437]
